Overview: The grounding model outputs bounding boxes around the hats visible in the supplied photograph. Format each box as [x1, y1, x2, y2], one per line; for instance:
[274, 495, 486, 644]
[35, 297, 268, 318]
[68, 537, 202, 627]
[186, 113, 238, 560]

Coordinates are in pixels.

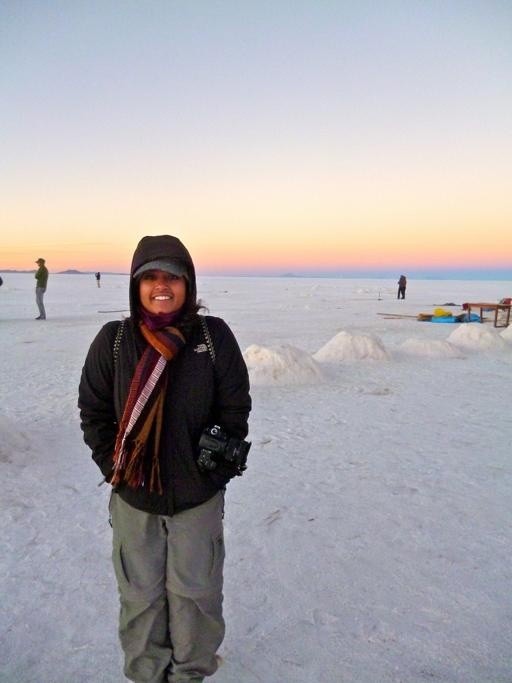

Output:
[35, 258, 44, 263]
[132, 259, 189, 281]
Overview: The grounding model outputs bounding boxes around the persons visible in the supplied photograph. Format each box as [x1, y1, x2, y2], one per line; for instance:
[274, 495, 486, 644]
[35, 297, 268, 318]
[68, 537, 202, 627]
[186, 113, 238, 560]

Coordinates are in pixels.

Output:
[401, 275, 407, 298]
[95, 271, 102, 288]
[77, 233, 253, 682]
[33, 257, 50, 320]
[397, 275, 404, 298]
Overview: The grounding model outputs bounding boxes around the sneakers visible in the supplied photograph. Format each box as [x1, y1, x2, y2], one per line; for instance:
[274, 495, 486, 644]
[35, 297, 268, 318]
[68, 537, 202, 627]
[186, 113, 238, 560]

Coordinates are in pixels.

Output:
[35, 316, 46, 320]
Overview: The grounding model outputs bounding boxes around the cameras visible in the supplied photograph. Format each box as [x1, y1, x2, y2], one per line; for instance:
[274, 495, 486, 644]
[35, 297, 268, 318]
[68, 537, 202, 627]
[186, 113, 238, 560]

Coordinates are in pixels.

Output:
[197, 423, 251, 476]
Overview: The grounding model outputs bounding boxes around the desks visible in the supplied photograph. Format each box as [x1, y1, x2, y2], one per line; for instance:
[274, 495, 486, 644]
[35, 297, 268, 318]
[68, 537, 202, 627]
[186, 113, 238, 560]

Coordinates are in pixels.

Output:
[462, 303, 512, 327]
